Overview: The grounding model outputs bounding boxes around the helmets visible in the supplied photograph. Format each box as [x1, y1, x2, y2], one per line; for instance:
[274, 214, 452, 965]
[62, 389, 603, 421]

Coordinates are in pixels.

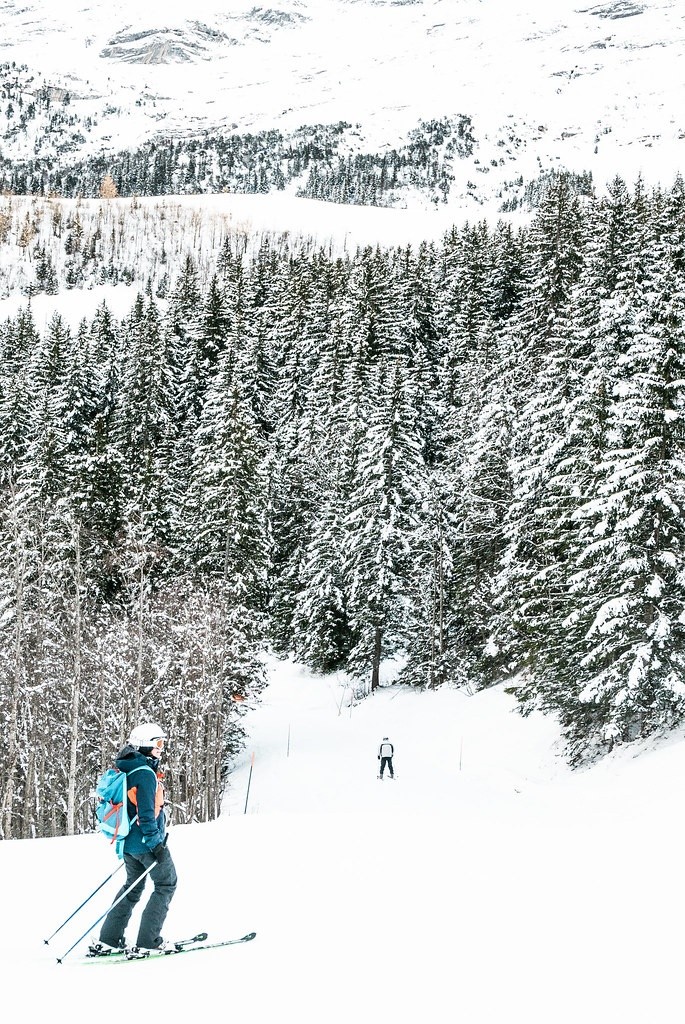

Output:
[131, 723, 166, 741]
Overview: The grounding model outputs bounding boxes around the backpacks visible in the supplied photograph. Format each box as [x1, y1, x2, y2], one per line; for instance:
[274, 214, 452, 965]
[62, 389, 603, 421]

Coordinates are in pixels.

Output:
[96, 765, 158, 840]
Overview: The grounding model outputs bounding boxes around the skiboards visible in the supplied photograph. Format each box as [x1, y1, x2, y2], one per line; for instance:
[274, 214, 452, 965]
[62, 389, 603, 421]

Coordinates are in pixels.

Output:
[65, 932, 257, 965]
[377, 774, 396, 780]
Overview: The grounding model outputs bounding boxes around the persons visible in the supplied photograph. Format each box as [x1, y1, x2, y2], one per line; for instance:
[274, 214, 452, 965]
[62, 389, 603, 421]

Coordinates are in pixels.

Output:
[378, 736, 395, 779]
[88, 722, 182, 959]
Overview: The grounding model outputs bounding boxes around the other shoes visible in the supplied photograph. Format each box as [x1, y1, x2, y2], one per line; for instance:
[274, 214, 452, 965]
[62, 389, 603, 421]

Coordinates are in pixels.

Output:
[136, 940, 175, 954]
[99, 941, 132, 954]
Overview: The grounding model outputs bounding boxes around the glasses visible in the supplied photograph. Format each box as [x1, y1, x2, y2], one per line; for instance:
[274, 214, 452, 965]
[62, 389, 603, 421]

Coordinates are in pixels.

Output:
[156, 739, 164, 749]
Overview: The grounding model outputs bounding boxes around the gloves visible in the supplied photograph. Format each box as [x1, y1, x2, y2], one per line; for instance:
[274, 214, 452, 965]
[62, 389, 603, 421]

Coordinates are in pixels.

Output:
[152, 842, 170, 865]
